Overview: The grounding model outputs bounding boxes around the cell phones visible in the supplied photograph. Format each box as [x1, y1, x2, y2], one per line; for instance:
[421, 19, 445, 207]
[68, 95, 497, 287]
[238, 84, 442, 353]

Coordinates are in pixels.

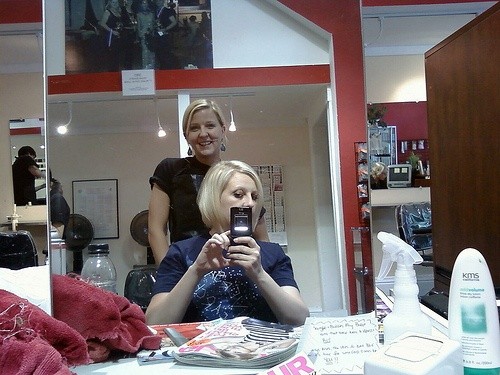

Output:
[229, 206, 253, 247]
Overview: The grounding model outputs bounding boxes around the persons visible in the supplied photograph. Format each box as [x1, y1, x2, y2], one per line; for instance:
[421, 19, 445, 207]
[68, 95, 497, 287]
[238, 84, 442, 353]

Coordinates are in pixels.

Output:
[148, 99, 270, 272]
[12, 146, 46, 206]
[371, 162, 389, 190]
[98, 0, 213, 69]
[145, 161, 307, 327]
[220, 325, 297, 361]
[49, 169, 70, 238]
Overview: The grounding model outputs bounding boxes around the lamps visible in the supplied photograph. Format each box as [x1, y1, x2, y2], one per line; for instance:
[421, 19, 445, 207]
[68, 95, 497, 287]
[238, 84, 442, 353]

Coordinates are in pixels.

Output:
[228, 96, 237, 132]
[57, 102, 74, 135]
[153, 99, 166, 138]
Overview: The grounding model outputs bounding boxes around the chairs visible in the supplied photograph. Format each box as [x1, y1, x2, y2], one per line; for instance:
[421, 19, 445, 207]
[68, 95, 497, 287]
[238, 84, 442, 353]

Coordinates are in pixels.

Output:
[395, 201, 433, 261]
[0, 230, 38, 270]
[124, 268, 159, 314]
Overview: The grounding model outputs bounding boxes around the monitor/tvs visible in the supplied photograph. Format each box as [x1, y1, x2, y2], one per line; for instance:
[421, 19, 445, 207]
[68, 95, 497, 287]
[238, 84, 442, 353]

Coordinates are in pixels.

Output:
[387, 164, 413, 188]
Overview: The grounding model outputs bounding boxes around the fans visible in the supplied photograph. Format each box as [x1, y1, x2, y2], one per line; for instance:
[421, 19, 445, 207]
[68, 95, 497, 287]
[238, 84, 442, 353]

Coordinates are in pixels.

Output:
[130, 210, 167, 264]
[63, 214, 94, 272]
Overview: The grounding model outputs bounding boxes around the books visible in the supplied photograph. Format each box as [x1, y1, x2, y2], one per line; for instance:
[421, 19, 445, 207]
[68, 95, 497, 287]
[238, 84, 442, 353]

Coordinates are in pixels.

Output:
[172, 315, 303, 369]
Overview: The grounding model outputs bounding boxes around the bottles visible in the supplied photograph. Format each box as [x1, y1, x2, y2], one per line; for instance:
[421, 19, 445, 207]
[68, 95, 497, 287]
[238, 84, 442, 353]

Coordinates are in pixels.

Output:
[80, 243, 117, 294]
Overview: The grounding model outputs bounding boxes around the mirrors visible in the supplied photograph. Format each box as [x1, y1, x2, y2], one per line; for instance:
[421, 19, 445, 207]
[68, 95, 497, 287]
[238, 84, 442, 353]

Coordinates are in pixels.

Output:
[10, 134, 46, 199]
[43, 85, 350, 324]
[361, 0, 500, 310]
